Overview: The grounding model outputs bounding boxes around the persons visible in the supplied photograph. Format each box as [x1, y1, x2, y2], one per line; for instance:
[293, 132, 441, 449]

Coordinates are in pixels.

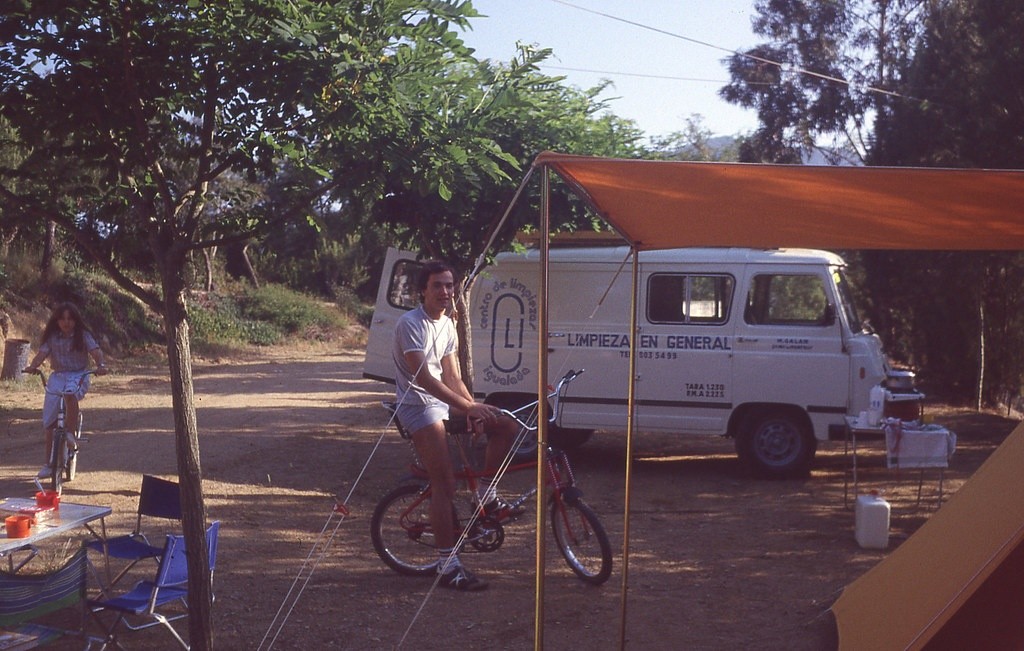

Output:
[393, 260, 525, 591]
[25, 302, 108, 478]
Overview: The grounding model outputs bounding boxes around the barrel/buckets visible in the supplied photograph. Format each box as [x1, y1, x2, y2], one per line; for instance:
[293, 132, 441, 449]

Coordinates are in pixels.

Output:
[854, 490, 891, 549]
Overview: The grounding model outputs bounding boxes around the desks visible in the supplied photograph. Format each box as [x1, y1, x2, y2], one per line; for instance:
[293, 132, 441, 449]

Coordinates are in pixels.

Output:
[845, 416, 948, 510]
[0, 497, 112, 586]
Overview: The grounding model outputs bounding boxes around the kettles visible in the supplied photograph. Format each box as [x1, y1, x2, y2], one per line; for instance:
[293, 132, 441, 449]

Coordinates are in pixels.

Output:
[883, 363, 916, 389]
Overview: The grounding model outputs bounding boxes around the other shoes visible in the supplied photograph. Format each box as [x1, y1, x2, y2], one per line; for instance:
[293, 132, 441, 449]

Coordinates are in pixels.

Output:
[38, 466, 53, 478]
[66, 433, 75, 446]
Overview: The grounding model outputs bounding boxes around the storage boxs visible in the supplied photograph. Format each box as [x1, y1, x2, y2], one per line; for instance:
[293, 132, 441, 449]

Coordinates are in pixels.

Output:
[883, 389, 925, 422]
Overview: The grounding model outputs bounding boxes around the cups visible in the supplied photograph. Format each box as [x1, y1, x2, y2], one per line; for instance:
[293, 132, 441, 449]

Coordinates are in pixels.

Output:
[36, 491, 60, 511]
[5, 515, 36, 538]
[856, 411, 868, 428]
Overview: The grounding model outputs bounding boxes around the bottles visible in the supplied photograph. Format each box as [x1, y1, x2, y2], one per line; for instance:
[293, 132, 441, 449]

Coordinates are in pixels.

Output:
[869, 382, 884, 425]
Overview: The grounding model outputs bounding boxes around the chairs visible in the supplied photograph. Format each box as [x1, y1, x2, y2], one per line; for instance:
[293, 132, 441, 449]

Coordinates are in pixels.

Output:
[85, 475, 216, 619]
[84, 521, 221, 651]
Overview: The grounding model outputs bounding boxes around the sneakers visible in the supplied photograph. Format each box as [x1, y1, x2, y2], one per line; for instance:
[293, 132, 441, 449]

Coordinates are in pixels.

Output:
[437, 563, 487, 591]
[471, 497, 525, 516]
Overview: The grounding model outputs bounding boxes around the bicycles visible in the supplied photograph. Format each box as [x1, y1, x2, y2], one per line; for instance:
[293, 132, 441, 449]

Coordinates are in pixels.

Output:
[366, 367, 616, 590]
[15, 363, 112, 501]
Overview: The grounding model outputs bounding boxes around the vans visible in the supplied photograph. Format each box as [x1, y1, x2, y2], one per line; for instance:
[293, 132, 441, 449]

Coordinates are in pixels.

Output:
[466, 244, 887, 476]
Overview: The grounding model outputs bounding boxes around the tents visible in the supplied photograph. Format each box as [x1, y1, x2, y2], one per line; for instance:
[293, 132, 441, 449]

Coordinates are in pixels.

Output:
[534, 150, 1024, 651]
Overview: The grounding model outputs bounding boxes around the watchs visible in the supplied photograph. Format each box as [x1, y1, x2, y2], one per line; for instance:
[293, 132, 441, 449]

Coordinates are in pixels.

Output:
[97, 363, 105, 368]
